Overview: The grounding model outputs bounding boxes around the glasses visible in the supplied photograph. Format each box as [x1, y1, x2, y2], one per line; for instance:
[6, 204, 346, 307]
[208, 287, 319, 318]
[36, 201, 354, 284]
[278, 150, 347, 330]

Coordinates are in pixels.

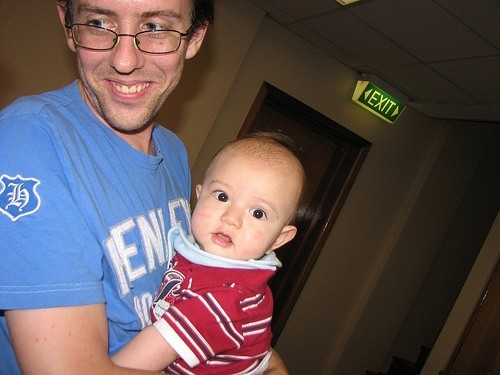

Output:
[65, 22, 195, 54]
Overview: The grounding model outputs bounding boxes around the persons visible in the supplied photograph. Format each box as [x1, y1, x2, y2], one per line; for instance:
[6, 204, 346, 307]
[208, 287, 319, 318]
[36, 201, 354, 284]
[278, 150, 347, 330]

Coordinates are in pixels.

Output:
[0, 0, 287, 375]
[111, 134, 307, 375]
